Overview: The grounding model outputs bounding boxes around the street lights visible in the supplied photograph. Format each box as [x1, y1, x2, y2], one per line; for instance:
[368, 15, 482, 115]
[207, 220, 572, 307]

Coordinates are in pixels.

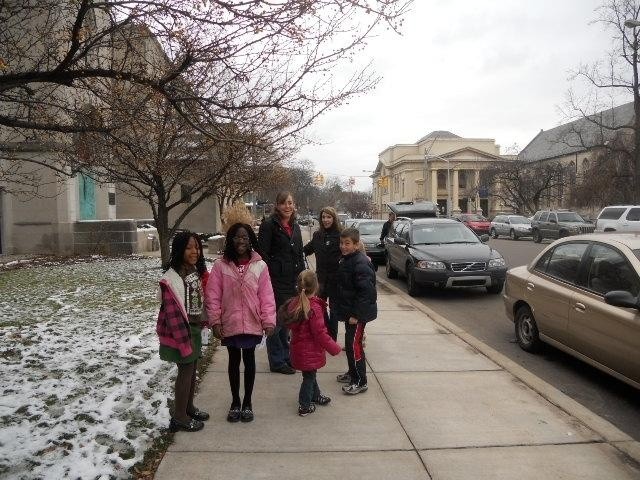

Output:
[362, 168, 376, 220]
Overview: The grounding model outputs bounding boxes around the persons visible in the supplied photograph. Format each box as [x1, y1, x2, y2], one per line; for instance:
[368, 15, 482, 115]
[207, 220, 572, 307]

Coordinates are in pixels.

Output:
[331, 229, 377, 396]
[157, 231, 211, 431]
[257, 192, 305, 375]
[380, 212, 395, 245]
[205, 222, 277, 423]
[301, 207, 344, 344]
[277, 269, 341, 415]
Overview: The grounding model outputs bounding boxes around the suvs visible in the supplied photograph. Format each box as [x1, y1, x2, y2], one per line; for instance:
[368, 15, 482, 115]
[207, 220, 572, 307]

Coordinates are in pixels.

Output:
[449, 203, 639, 250]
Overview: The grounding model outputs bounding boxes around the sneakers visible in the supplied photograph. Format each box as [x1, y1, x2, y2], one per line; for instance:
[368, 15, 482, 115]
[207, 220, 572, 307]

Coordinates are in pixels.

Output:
[337, 369, 368, 396]
[271, 361, 297, 376]
[297, 392, 331, 417]
[226, 398, 254, 422]
[169, 405, 210, 433]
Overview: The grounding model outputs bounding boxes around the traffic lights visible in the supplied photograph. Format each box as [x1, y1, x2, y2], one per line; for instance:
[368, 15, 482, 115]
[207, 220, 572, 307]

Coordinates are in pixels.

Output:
[379, 177, 383, 189]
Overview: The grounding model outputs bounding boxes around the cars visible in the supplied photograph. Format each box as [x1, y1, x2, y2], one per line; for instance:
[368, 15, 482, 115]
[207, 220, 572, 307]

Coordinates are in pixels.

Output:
[382, 197, 509, 299]
[289, 209, 396, 270]
[501, 232, 640, 398]
